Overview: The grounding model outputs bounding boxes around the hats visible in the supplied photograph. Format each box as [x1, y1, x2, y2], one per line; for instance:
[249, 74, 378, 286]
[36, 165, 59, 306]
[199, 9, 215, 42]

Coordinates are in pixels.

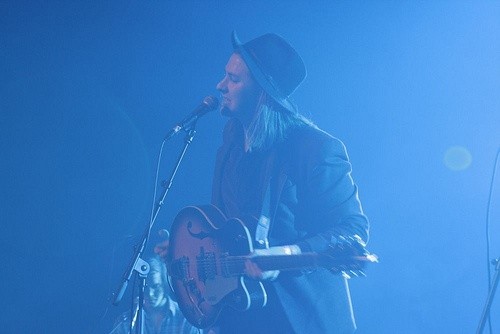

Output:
[230, 27, 307, 118]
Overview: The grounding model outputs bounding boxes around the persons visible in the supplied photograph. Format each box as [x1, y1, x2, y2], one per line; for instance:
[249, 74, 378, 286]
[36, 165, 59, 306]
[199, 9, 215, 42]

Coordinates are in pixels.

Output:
[152, 30, 370, 334]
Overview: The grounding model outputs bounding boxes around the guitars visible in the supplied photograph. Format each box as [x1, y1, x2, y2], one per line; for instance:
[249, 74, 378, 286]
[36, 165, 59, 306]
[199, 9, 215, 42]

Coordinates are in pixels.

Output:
[167, 204, 379, 329]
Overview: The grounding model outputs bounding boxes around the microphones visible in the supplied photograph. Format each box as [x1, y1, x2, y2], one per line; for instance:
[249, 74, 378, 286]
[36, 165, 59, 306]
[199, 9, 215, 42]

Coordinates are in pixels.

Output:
[165, 96, 219, 140]
